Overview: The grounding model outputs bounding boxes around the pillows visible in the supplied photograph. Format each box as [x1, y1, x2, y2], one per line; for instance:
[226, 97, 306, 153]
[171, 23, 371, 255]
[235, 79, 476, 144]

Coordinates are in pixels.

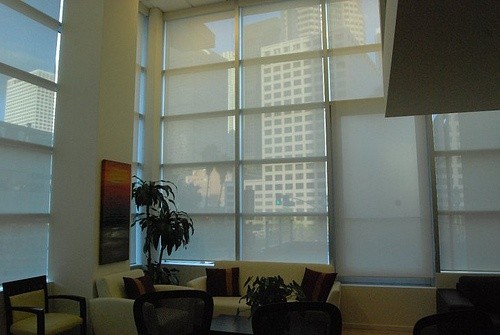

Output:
[204, 267, 240, 297]
[295, 267, 338, 303]
[123, 273, 153, 300]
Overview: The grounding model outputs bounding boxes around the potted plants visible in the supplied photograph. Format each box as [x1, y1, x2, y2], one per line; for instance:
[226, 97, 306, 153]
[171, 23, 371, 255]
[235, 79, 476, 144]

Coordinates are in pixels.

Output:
[237, 275, 306, 319]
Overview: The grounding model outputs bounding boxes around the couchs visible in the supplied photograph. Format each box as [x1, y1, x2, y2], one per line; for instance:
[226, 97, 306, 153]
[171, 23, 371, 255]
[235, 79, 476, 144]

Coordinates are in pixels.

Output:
[89, 269, 204, 335]
[187, 261, 341, 318]
[437, 275, 500, 335]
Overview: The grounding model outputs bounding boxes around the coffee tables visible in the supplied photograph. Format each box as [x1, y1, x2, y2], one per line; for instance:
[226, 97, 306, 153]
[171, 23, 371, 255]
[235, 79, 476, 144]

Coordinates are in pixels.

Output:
[209, 314, 253, 335]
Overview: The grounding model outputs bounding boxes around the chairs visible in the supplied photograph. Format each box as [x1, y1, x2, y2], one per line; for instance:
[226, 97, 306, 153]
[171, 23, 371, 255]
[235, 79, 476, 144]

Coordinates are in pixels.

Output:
[413, 309, 494, 335]
[2, 275, 86, 335]
[252, 302, 343, 335]
[133, 290, 214, 335]
